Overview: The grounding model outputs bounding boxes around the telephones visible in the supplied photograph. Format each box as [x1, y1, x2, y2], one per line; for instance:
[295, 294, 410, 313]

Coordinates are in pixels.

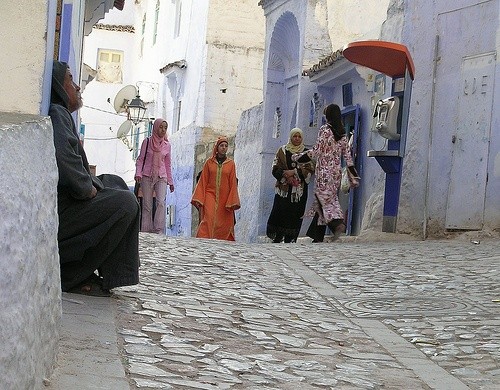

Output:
[372, 96, 402, 140]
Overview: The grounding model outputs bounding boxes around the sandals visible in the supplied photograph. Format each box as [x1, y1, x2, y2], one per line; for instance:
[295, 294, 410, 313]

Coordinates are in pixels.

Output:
[65, 271, 114, 297]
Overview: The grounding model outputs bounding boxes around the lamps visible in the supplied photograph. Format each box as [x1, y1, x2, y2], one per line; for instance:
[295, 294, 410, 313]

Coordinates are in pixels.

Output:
[127, 96, 145, 124]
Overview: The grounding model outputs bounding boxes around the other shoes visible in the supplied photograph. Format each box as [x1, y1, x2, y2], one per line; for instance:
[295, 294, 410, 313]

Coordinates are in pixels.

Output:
[331, 222, 346, 242]
[312, 238, 324, 243]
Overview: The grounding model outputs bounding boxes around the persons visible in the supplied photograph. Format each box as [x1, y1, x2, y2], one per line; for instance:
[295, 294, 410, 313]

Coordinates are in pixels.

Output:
[48, 60, 141, 299]
[190, 137, 241, 242]
[135, 118, 175, 234]
[291, 103, 363, 243]
[266, 127, 315, 244]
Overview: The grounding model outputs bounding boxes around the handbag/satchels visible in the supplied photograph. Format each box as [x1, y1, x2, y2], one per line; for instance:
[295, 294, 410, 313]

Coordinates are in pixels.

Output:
[134, 182, 140, 196]
